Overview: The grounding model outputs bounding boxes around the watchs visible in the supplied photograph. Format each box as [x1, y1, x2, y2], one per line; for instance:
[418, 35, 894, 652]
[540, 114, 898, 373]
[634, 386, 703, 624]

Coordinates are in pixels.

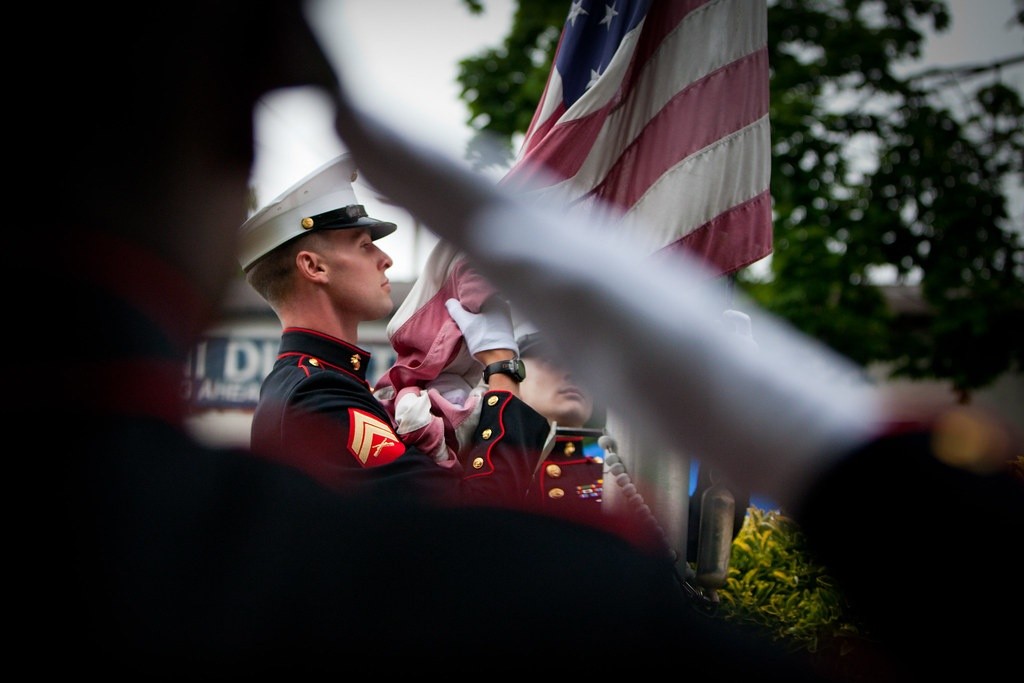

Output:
[483, 358, 526, 384]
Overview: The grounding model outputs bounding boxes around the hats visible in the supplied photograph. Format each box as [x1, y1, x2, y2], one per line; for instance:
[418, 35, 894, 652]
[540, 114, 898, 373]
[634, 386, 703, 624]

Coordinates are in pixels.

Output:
[503, 299, 546, 359]
[233, 150, 398, 275]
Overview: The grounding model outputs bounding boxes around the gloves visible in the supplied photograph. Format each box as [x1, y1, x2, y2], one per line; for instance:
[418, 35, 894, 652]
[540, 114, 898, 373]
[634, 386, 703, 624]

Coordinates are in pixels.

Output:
[445, 297, 520, 362]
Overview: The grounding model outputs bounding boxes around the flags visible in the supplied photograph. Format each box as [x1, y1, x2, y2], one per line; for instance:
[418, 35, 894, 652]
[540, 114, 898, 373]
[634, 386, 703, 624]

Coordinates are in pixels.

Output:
[372, 0, 773, 473]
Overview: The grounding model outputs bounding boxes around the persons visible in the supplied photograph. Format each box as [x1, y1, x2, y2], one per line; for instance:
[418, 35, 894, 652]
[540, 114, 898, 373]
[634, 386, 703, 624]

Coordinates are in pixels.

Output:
[0, 0, 1024, 682]
[507, 310, 784, 562]
[233, 149, 551, 516]
